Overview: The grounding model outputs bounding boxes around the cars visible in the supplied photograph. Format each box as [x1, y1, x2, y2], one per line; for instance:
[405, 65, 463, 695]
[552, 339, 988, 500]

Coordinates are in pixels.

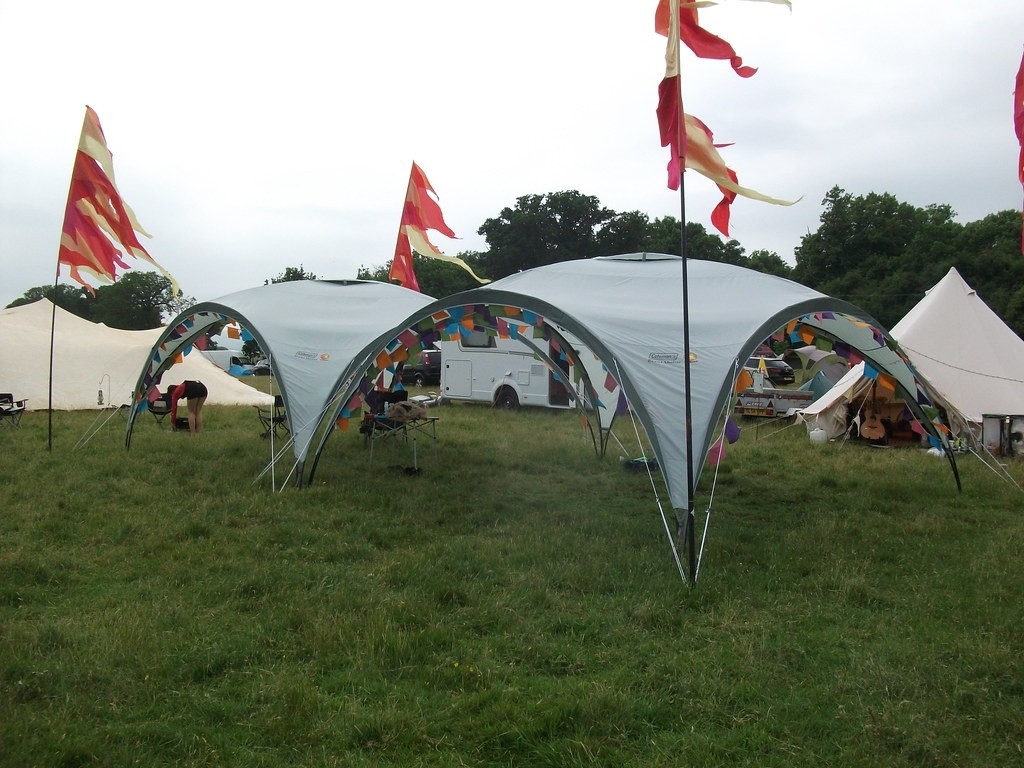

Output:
[254, 359, 275, 376]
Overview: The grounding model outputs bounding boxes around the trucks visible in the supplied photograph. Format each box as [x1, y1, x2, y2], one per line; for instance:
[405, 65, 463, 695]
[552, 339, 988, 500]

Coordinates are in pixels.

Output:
[200, 349, 255, 378]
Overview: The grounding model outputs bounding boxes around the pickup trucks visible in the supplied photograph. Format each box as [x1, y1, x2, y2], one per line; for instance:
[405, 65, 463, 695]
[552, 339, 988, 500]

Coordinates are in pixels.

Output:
[399, 350, 441, 387]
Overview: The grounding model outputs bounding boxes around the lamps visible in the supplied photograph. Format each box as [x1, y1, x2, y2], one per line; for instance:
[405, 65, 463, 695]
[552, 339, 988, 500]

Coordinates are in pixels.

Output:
[96, 383, 104, 405]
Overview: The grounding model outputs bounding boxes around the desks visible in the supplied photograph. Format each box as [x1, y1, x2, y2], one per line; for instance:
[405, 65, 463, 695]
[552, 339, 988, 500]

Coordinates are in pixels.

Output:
[367, 417, 440, 472]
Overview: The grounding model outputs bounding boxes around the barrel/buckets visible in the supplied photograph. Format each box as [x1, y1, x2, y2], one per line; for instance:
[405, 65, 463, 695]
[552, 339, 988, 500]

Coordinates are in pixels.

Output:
[809, 428, 827, 446]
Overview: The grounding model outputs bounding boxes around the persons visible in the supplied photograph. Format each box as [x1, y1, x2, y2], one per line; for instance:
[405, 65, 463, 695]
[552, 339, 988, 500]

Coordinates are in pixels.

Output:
[167, 380, 208, 433]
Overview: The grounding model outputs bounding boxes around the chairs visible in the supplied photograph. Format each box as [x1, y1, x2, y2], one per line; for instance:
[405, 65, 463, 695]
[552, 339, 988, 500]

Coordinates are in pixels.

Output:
[0, 392, 29, 429]
[252, 395, 291, 442]
[148, 393, 172, 430]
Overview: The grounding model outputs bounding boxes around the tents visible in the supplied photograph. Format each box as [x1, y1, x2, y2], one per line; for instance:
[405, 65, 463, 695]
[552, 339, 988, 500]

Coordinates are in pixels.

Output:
[0, 251, 1024, 583]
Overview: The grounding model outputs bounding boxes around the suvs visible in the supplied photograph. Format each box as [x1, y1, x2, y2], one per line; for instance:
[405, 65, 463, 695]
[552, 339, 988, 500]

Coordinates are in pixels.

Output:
[744, 357, 795, 386]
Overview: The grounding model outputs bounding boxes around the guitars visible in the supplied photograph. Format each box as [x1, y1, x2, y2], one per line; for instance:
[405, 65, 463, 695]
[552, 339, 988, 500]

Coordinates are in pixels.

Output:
[860, 377, 888, 441]
